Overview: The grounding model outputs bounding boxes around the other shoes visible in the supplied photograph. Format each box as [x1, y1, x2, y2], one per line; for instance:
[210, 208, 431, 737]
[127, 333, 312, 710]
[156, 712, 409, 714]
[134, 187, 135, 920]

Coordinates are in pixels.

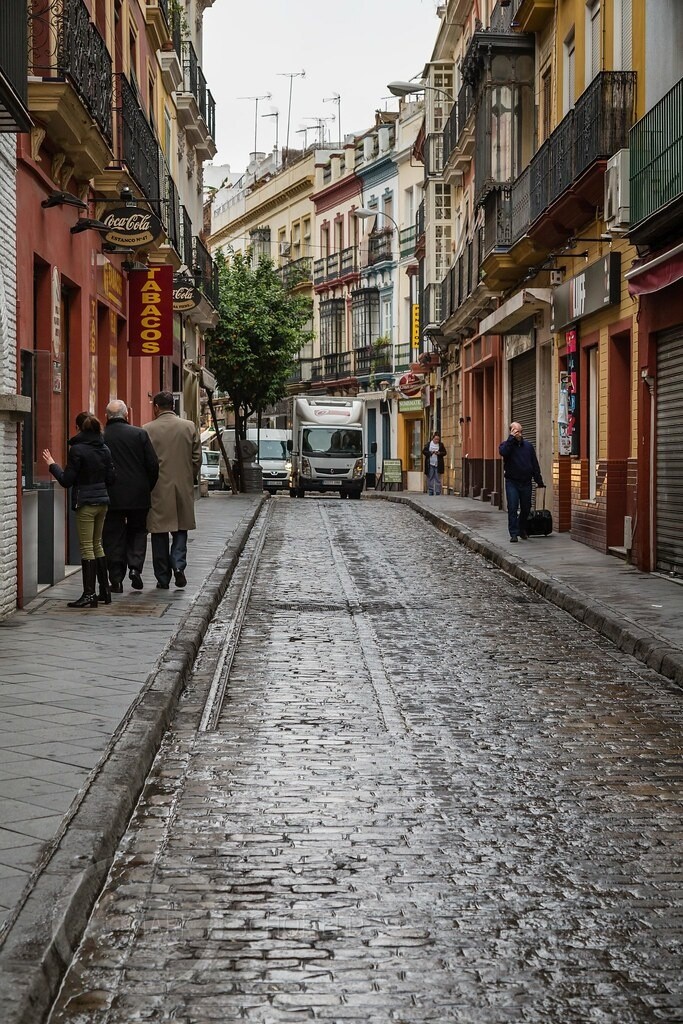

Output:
[520, 531, 528, 539]
[156, 575, 169, 589]
[174, 569, 187, 587]
[436, 492, 440, 495]
[510, 536, 518, 542]
[429, 492, 433, 495]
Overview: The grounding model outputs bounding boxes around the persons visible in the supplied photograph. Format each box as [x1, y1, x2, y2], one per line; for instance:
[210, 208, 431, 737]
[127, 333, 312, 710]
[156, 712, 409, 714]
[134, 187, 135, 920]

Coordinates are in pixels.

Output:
[142, 392, 202, 589]
[500, 423, 545, 543]
[42, 411, 114, 607]
[422, 431, 447, 496]
[103, 400, 159, 593]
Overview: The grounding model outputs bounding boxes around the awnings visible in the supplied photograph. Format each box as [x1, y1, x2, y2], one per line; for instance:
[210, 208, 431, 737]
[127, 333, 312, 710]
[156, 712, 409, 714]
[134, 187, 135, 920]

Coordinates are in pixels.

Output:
[199, 428, 224, 445]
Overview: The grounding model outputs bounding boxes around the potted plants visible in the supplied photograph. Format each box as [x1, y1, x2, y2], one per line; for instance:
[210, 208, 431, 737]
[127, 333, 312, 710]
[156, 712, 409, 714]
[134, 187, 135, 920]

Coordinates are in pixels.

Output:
[162, 0, 192, 51]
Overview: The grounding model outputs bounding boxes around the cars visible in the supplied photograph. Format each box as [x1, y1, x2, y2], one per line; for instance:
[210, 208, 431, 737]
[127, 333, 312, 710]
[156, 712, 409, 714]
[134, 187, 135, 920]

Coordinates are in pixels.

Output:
[193, 450, 223, 491]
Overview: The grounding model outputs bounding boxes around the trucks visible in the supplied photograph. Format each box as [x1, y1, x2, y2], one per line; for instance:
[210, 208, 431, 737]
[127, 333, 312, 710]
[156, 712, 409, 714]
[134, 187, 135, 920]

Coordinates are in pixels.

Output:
[287, 394, 377, 499]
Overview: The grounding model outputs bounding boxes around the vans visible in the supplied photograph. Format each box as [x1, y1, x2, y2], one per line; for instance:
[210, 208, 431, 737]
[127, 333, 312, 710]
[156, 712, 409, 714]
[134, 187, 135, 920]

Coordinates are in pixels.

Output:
[218, 428, 293, 495]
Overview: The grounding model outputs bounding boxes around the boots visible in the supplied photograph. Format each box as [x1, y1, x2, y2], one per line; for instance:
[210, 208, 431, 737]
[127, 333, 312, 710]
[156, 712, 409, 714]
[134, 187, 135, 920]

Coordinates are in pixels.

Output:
[67, 559, 98, 608]
[95, 555, 112, 604]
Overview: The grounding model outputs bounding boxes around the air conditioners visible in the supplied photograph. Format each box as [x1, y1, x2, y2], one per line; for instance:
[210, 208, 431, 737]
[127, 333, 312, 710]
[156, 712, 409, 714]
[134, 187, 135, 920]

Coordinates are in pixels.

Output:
[603, 149, 630, 230]
[279, 241, 290, 255]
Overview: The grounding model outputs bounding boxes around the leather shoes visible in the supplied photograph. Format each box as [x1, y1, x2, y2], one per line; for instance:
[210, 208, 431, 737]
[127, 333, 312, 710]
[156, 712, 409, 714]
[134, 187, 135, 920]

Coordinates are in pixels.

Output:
[110, 583, 123, 593]
[129, 570, 143, 589]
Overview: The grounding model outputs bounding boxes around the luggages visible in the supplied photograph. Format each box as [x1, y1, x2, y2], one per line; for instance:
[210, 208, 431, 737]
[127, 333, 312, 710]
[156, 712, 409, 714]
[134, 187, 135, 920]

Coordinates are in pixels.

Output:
[521, 486, 552, 537]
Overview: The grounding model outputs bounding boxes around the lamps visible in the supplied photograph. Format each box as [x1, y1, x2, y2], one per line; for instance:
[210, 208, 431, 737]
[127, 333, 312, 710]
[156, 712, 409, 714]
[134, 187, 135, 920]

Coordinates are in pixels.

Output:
[528, 266, 566, 277]
[102, 243, 136, 254]
[546, 249, 588, 263]
[41, 192, 90, 210]
[121, 261, 149, 272]
[70, 218, 112, 234]
[565, 237, 613, 246]
[353, 208, 399, 233]
[386, 81, 455, 101]
